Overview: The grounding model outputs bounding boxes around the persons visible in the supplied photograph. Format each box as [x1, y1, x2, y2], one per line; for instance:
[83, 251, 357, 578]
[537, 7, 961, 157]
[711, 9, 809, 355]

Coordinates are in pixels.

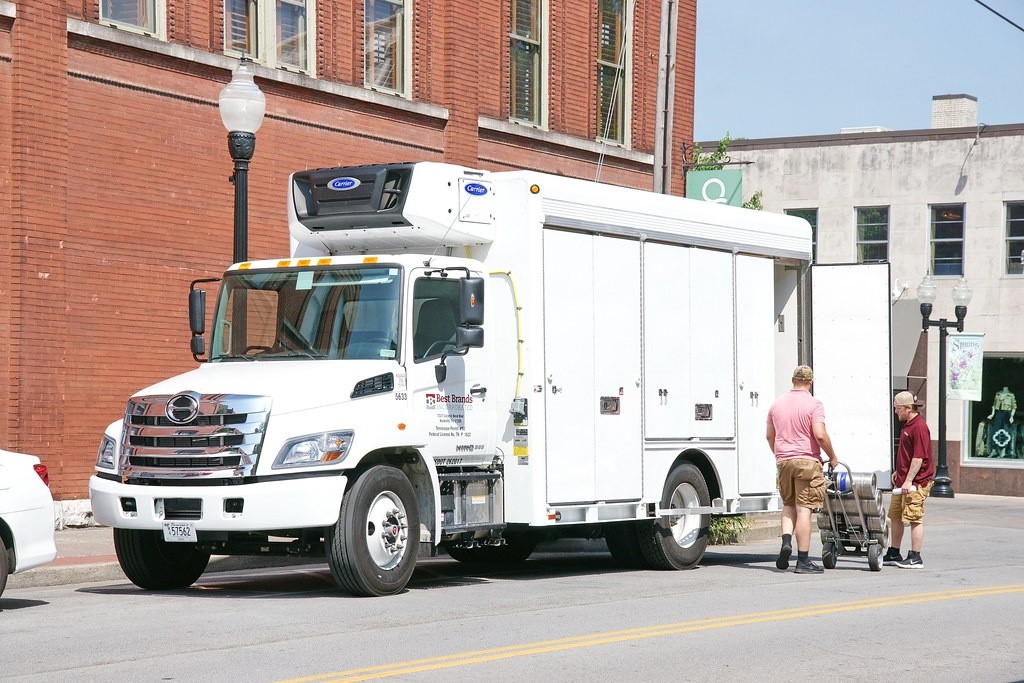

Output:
[987, 387, 1017, 459]
[883, 391, 935, 569]
[766, 364, 838, 574]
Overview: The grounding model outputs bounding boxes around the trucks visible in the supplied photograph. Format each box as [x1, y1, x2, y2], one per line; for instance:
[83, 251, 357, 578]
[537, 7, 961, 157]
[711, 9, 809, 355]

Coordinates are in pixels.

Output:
[86, 159, 896, 599]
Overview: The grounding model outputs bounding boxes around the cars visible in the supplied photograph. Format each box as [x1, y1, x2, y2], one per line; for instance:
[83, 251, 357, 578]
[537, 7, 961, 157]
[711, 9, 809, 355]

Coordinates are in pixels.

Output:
[0, 448, 56, 599]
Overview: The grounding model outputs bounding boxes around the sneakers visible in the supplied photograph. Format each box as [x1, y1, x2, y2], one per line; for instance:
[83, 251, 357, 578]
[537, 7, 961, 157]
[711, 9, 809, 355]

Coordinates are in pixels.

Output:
[776, 542, 792, 570]
[794, 560, 824, 574]
[883, 547, 903, 565]
[894, 550, 923, 569]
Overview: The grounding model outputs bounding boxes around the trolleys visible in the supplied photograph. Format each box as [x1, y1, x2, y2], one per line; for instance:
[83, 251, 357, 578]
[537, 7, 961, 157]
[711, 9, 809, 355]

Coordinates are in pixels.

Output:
[812, 461, 889, 571]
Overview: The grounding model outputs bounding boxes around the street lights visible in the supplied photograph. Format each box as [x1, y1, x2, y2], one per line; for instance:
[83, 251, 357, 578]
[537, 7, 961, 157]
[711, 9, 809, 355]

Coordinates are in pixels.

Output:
[914, 266, 972, 500]
[218, 59, 266, 349]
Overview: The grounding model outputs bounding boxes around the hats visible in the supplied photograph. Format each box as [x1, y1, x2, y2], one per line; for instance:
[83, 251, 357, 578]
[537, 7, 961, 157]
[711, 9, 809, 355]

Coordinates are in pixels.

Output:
[793, 365, 813, 380]
[894, 391, 925, 406]
